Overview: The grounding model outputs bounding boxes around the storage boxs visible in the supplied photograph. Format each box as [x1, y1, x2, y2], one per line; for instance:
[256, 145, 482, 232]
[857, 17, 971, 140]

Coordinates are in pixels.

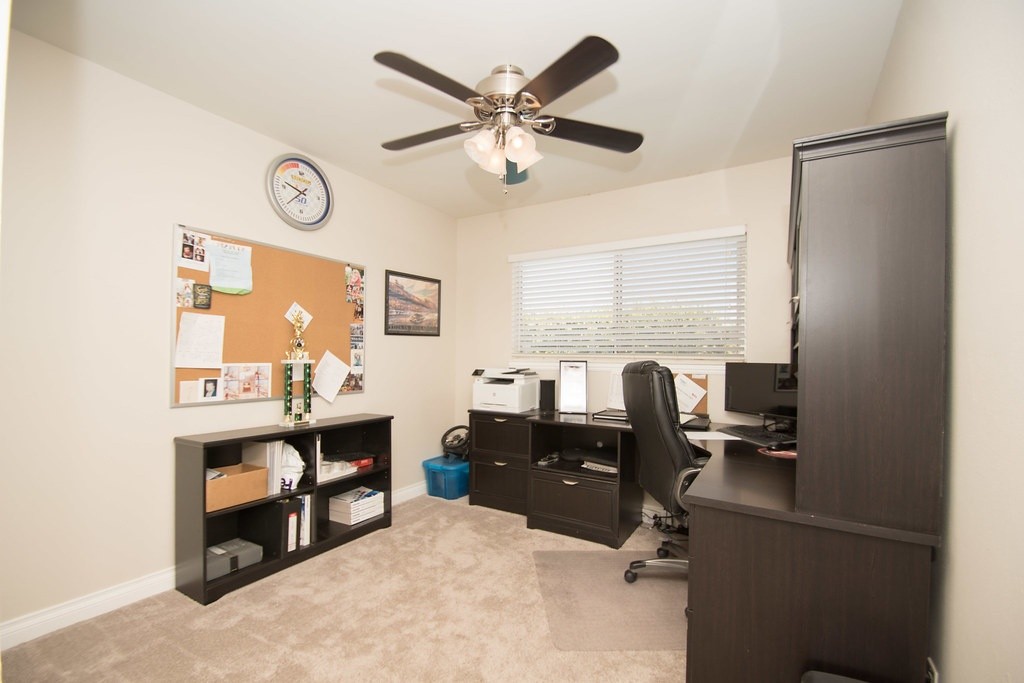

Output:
[205, 463, 269, 513]
[422, 453, 470, 501]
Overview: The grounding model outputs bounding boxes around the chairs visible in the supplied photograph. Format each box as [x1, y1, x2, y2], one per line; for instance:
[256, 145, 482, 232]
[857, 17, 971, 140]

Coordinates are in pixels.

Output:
[621, 359, 714, 584]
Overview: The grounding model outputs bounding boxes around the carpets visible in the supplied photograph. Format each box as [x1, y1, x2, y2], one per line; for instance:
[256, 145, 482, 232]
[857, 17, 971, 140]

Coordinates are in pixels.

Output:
[534, 550, 689, 652]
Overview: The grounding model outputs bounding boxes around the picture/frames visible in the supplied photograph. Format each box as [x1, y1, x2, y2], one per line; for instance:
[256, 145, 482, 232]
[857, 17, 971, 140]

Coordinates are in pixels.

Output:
[385, 269, 441, 338]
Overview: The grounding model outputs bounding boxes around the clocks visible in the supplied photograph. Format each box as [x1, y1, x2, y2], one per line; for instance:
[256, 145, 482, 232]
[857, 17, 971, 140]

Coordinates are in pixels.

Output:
[266, 153, 335, 230]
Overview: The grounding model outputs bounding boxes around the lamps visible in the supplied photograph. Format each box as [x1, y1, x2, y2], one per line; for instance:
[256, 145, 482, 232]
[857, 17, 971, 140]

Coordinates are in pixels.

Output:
[463, 111, 543, 196]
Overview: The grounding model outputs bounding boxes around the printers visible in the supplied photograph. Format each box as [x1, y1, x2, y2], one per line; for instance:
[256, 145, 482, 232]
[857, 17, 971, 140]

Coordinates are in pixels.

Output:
[472, 368, 540, 413]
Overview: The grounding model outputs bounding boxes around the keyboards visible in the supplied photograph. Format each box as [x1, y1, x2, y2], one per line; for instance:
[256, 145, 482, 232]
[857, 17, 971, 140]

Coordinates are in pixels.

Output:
[716, 425, 797, 448]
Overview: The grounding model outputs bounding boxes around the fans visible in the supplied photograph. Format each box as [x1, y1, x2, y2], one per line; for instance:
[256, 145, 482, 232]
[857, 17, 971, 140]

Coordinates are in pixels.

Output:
[373, 35, 644, 186]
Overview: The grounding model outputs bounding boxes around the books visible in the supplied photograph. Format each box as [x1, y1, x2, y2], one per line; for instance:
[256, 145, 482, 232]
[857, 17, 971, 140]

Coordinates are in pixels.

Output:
[330, 486, 384, 525]
[592, 409, 629, 421]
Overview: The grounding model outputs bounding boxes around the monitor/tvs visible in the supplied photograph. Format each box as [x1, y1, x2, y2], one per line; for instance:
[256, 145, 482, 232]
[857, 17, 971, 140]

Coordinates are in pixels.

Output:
[725, 363, 798, 434]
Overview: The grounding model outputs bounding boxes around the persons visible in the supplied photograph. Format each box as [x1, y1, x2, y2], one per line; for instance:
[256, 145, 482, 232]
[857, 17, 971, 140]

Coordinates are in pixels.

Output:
[205, 381, 216, 396]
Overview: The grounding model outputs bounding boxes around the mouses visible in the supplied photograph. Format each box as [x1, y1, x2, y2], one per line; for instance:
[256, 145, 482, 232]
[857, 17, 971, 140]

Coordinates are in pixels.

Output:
[767, 442, 789, 451]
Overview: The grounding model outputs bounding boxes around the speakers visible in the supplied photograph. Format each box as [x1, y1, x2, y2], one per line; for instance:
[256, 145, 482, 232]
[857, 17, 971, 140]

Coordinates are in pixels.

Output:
[540, 379, 555, 415]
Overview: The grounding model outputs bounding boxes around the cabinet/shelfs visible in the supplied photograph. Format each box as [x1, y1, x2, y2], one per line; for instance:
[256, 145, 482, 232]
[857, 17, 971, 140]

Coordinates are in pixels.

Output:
[174, 412, 394, 606]
[685, 122, 949, 683]
[469, 412, 538, 516]
[527, 418, 643, 551]
